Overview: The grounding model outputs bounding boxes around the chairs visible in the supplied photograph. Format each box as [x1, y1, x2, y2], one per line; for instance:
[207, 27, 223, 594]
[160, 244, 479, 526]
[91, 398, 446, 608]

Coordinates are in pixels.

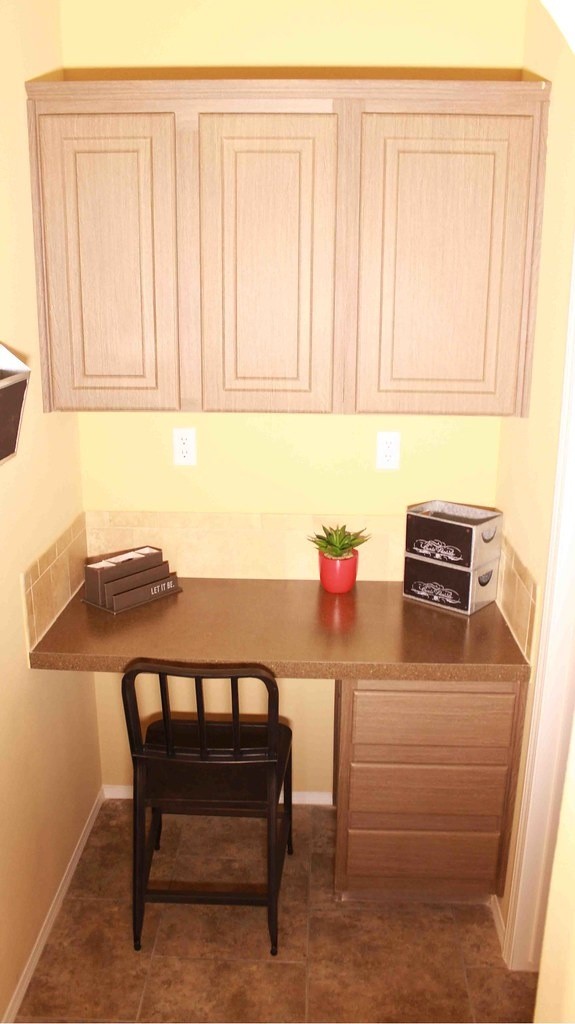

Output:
[121, 657, 294, 956]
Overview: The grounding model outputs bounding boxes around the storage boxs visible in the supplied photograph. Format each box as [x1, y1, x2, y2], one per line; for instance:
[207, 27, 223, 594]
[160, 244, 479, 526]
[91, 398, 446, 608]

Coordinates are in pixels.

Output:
[405, 500, 503, 571]
[402, 554, 503, 616]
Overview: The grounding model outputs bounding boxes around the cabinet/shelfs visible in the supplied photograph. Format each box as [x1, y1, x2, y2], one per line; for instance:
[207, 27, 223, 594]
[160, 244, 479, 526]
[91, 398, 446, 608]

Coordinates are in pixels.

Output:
[25, 67, 550, 421]
[27, 580, 531, 905]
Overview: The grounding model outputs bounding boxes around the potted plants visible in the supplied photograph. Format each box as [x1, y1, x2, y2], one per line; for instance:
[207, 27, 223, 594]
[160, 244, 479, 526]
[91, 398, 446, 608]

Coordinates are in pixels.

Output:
[308, 524, 371, 593]
